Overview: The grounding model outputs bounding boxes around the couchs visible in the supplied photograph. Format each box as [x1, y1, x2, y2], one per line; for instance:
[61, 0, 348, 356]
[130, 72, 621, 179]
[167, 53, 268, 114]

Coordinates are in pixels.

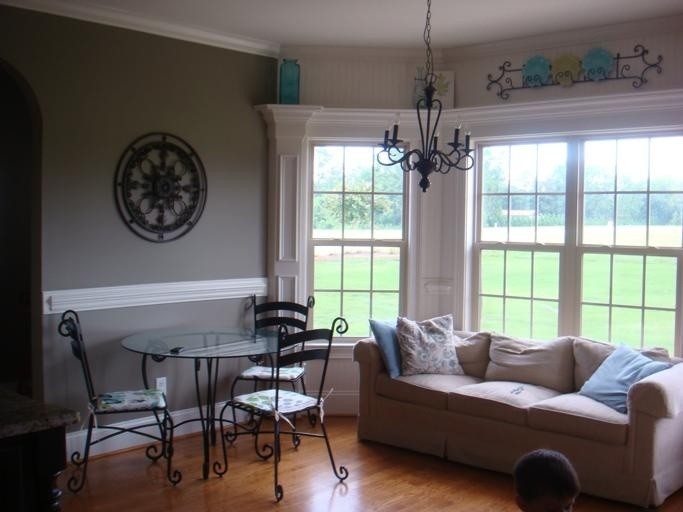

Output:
[351, 328, 682, 510]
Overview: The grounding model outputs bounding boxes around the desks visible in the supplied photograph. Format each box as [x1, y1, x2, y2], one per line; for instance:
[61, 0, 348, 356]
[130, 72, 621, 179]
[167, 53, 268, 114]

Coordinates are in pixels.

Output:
[118, 323, 299, 481]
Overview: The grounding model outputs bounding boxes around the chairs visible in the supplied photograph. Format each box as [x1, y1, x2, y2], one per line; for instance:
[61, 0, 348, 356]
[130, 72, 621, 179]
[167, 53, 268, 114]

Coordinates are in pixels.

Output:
[224, 292, 318, 448]
[211, 316, 348, 503]
[57, 310, 182, 493]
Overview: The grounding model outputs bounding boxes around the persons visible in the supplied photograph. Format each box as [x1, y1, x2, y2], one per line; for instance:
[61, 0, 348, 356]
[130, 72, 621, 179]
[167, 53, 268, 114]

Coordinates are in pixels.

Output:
[509, 446, 582, 512]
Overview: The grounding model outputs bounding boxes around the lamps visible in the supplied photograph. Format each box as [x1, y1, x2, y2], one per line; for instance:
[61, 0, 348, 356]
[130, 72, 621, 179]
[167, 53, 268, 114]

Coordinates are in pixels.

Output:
[374, 1, 477, 194]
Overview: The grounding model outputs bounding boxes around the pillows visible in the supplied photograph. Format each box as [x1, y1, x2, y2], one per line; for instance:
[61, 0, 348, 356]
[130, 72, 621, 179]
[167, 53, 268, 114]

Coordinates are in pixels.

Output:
[393, 315, 466, 376]
[578, 342, 673, 415]
[367, 318, 405, 379]
